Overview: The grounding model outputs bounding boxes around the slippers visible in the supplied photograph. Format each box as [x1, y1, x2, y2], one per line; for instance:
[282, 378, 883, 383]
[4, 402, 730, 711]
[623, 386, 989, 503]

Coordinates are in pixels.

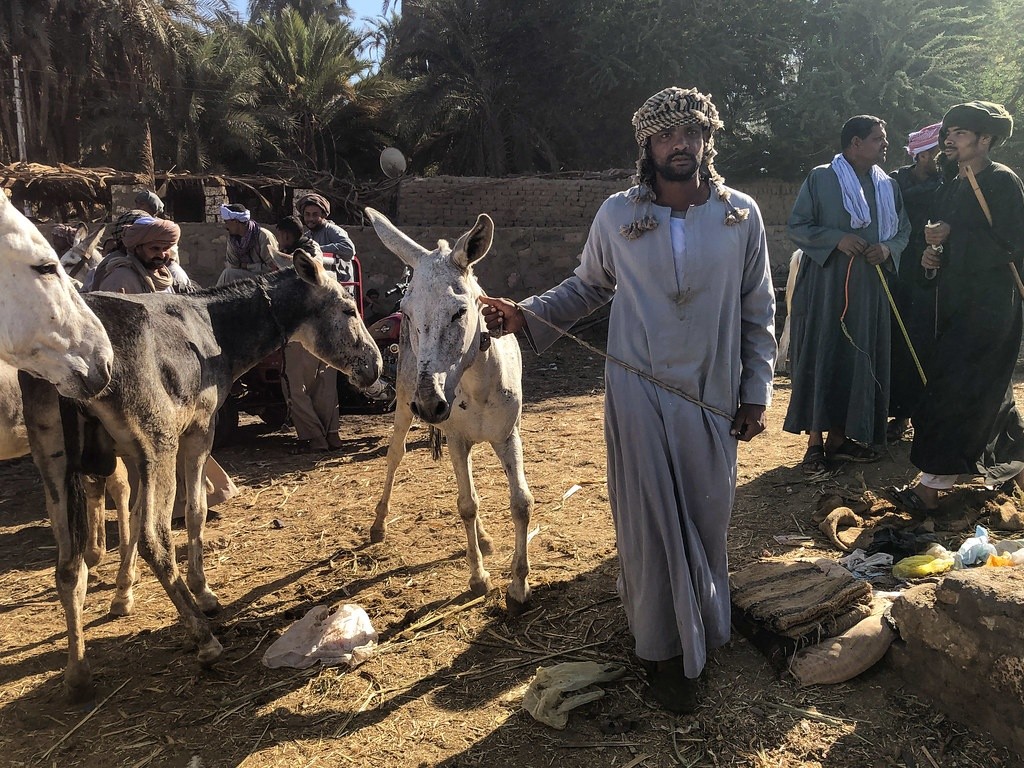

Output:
[281, 441, 329, 455]
[998, 480, 1019, 497]
[801, 444, 827, 475]
[826, 438, 882, 463]
[885, 487, 938, 520]
[326, 438, 340, 450]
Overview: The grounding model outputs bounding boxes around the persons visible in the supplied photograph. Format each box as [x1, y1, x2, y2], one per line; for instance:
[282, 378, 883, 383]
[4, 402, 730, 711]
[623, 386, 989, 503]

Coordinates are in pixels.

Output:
[79, 191, 238, 522]
[480, 86, 778, 713]
[783, 100, 1024, 514]
[216, 195, 354, 451]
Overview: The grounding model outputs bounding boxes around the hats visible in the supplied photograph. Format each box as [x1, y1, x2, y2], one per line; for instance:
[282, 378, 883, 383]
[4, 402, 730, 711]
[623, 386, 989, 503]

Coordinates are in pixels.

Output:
[111, 209, 152, 248]
[122, 217, 181, 248]
[135, 190, 164, 217]
[941, 100, 1013, 150]
[619, 86, 750, 241]
[907, 122, 943, 162]
[295, 194, 330, 218]
[220, 204, 250, 222]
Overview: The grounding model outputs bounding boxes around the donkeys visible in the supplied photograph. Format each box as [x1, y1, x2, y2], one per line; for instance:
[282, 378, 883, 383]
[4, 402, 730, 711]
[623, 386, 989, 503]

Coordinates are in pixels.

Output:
[363, 205, 538, 612]
[16, 250, 385, 702]
[0, 359, 137, 583]
[0, 187, 113, 400]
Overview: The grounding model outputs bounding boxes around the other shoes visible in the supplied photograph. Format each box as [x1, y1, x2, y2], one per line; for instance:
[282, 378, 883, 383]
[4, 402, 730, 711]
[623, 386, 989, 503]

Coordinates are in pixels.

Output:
[887, 419, 914, 442]
[638, 657, 696, 713]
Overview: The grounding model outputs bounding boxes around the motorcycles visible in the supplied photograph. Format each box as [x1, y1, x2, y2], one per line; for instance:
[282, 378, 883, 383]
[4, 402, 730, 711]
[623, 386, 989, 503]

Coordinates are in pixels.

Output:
[210, 251, 413, 449]
